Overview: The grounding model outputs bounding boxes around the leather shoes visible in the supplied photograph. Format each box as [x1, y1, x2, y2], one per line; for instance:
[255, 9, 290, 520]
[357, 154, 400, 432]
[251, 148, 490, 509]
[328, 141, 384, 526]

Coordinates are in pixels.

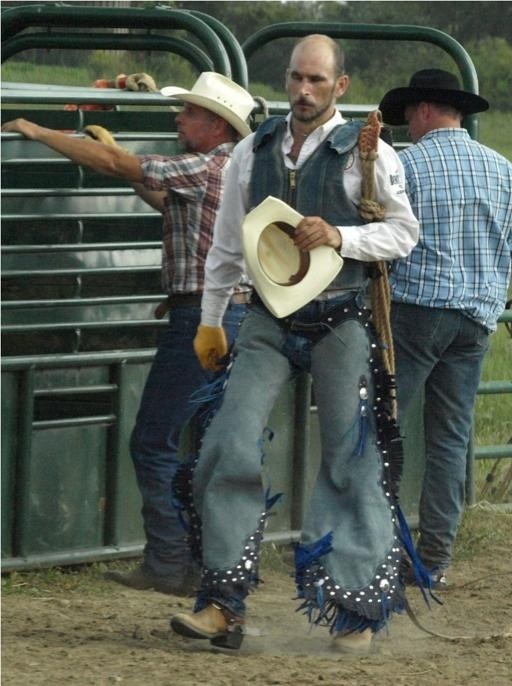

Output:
[333, 628, 371, 654]
[104, 562, 199, 598]
[402, 562, 443, 587]
[171, 603, 245, 650]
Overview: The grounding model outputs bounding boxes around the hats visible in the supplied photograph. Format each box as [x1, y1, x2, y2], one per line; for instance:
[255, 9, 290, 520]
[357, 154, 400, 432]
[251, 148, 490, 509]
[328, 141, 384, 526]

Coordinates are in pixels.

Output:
[160, 72, 257, 138]
[242, 195, 343, 320]
[378, 68, 489, 126]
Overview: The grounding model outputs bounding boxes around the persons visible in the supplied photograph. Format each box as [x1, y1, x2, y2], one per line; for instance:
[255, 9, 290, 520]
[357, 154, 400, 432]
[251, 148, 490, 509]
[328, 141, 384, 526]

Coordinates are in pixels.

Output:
[377, 68, 510, 592]
[170, 29, 420, 653]
[5, 66, 259, 605]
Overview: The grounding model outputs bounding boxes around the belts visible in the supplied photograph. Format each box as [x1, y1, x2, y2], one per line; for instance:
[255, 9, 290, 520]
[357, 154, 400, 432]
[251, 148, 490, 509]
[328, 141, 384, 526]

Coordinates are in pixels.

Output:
[154, 294, 250, 320]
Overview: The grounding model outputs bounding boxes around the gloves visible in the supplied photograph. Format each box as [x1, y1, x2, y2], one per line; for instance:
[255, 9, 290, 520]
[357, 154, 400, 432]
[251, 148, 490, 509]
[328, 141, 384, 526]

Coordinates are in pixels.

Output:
[194, 326, 227, 372]
[83, 125, 120, 153]
[114, 72, 154, 91]
